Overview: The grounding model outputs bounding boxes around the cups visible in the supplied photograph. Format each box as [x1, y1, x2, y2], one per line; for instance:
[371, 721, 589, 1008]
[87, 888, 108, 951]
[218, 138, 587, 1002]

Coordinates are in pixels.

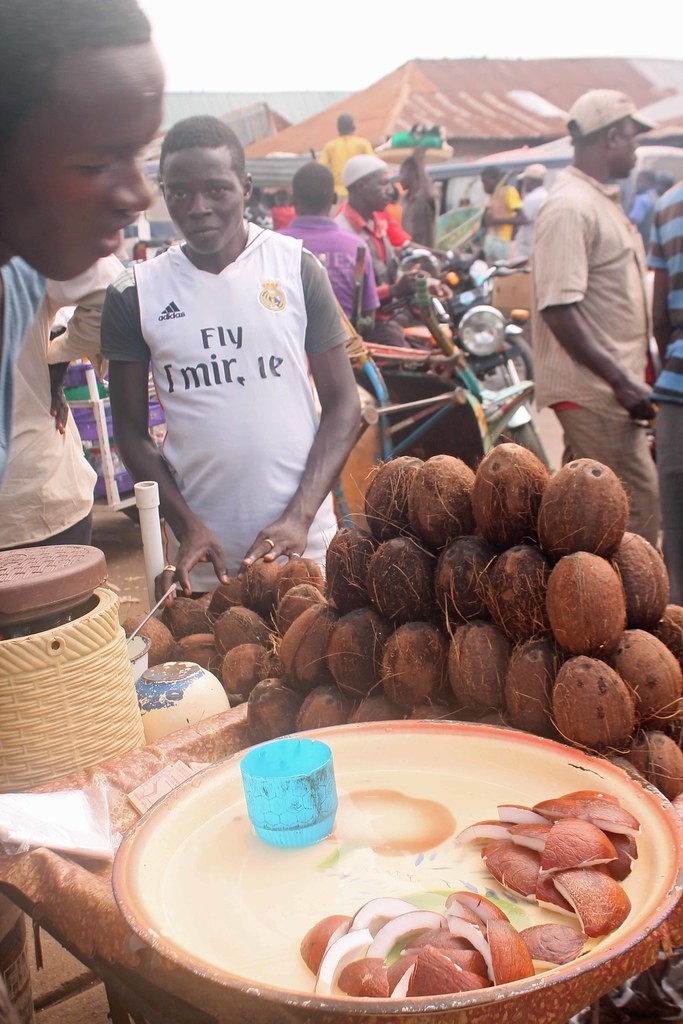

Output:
[241, 739, 338, 848]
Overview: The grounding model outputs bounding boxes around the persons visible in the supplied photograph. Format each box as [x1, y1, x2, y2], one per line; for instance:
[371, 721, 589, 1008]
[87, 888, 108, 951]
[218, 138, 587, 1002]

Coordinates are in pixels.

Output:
[515, 164, 549, 255]
[646, 182, 683, 607]
[276, 162, 381, 332]
[0, 252, 125, 546]
[101, 116, 362, 609]
[244, 186, 270, 229]
[481, 166, 527, 262]
[268, 191, 295, 231]
[398, 137, 436, 248]
[334, 155, 426, 306]
[531, 89, 659, 545]
[0, 0, 166, 475]
[320, 114, 372, 204]
[628, 171, 659, 257]
[653, 175, 672, 196]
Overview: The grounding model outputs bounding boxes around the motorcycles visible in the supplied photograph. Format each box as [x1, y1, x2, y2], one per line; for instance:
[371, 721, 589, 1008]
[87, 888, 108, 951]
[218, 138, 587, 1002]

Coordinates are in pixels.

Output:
[354, 248, 555, 476]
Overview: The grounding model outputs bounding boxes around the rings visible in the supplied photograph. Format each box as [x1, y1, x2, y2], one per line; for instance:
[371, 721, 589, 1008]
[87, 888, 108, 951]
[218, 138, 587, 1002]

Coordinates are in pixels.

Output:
[163, 565, 176, 571]
[292, 553, 300, 557]
[266, 539, 275, 548]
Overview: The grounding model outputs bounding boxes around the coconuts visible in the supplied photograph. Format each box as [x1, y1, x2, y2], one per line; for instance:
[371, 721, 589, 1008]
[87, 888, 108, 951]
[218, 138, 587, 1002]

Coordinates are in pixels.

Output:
[112, 442, 682, 803]
